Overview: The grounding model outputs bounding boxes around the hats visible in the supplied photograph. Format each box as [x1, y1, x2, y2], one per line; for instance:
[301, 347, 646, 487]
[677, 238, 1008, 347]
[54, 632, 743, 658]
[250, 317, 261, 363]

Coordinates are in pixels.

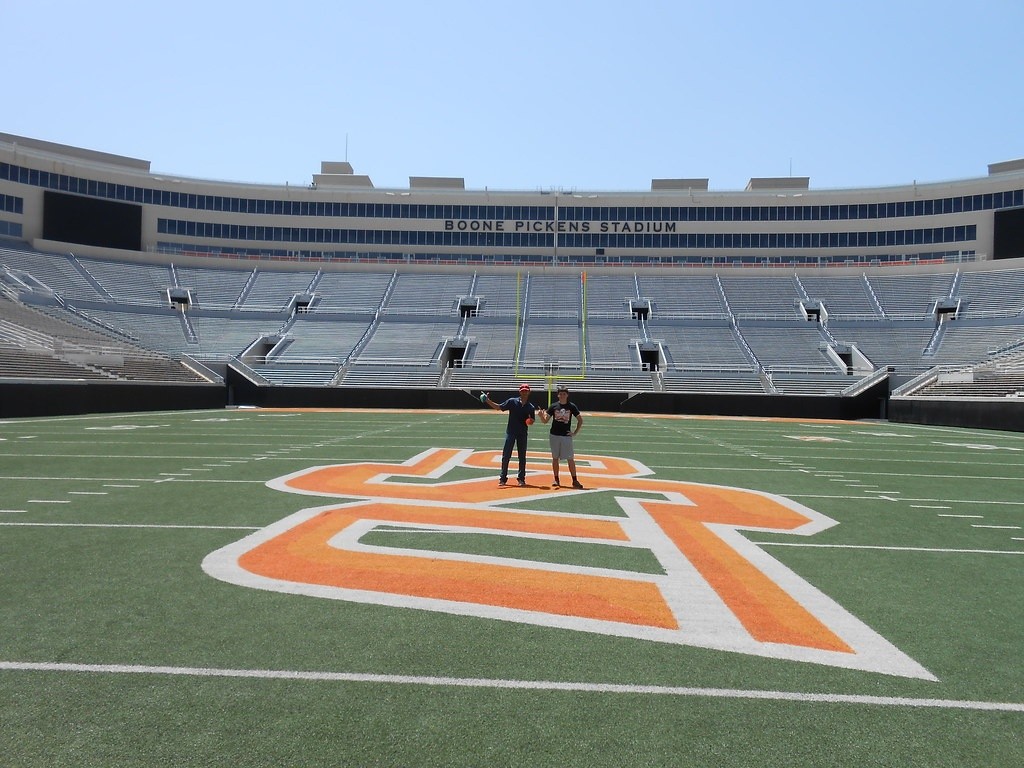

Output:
[519, 384, 530, 392]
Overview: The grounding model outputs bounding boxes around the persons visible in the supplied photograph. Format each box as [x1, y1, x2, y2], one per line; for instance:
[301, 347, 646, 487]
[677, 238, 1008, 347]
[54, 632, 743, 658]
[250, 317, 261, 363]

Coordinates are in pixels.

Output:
[537, 388, 584, 488]
[480, 384, 536, 486]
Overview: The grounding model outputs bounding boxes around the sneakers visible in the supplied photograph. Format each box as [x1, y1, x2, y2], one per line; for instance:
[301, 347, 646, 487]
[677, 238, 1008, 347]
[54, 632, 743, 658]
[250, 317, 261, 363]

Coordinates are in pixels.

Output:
[573, 480, 583, 488]
[518, 479, 525, 486]
[552, 481, 560, 485]
[499, 480, 505, 485]
